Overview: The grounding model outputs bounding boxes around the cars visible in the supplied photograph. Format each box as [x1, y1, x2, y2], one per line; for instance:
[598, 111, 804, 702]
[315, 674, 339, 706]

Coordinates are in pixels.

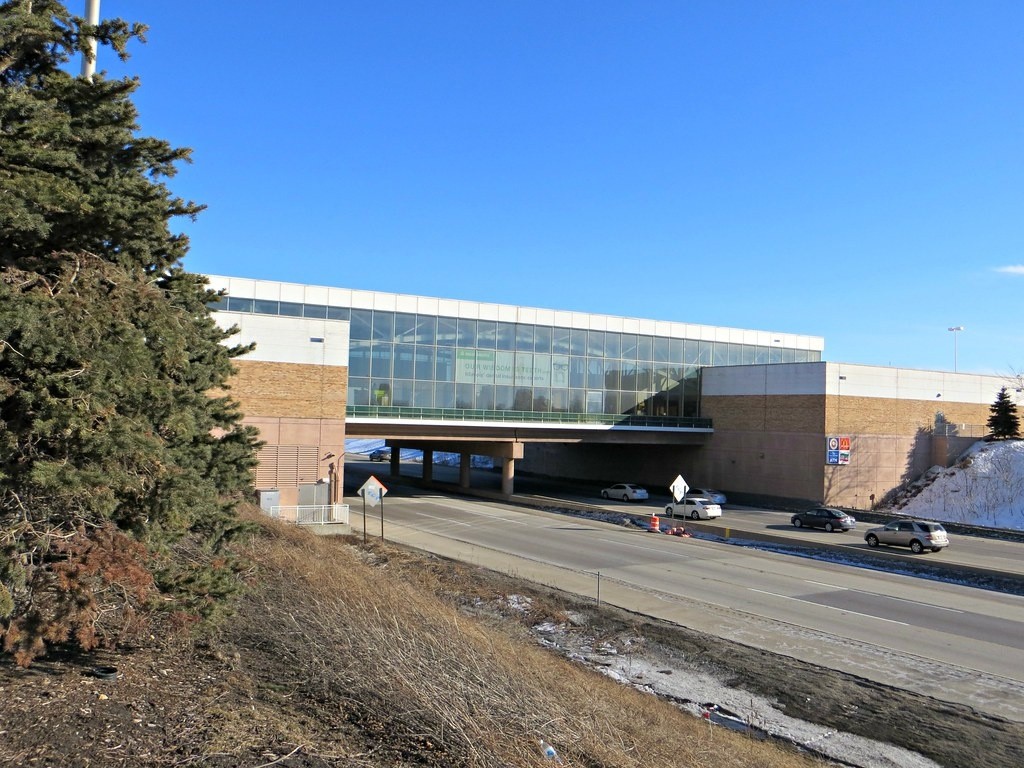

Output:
[665, 497, 722, 520]
[791, 507, 856, 533]
[602, 484, 649, 501]
[685, 488, 727, 505]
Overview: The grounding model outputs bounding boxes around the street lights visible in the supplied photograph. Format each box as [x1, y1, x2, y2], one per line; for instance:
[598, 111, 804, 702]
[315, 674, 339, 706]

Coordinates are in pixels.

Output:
[949, 327, 964, 372]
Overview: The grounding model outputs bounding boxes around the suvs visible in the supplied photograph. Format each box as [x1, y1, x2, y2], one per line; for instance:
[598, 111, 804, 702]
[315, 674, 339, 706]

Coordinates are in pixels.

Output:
[370, 450, 391, 462]
[864, 518, 950, 554]
